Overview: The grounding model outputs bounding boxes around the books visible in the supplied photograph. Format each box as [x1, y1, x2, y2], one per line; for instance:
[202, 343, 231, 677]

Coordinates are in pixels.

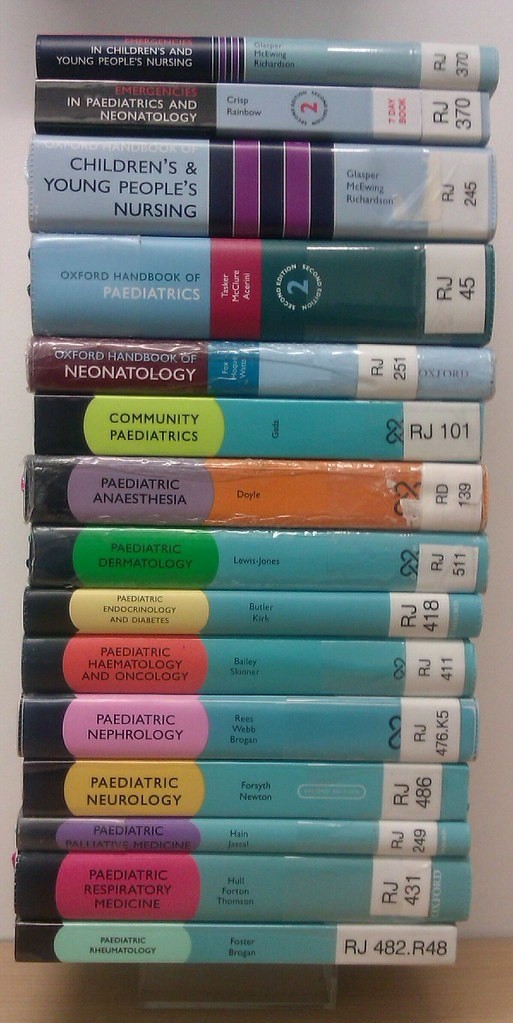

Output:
[21, 587, 486, 639]
[21, 753, 473, 824]
[24, 337, 496, 403]
[9, 916, 457, 967]
[20, 454, 490, 536]
[24, 526, 491, 595]
[30, 392, 487, 462]
[9, 852, 474, 923]
[35, 30, 500, 99]
[32, 79, 492, 148]
[27, 231, 496, 348]
[16, 692, 481, 764]
[16, 809, 473, 858]
[25, 133, 500, 241]
[20, 636, 476, 699]
